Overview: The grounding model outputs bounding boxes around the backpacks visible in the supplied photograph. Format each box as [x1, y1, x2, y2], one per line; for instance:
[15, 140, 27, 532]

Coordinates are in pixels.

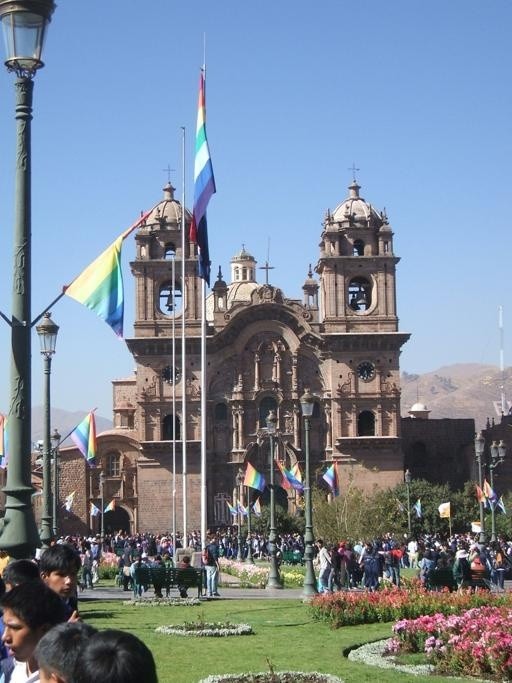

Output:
[202, 546, 210, 565]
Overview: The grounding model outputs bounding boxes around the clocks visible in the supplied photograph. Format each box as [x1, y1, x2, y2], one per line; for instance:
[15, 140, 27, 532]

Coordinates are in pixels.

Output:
[162, 365, 180, 384]
[355, 359, 378, 383]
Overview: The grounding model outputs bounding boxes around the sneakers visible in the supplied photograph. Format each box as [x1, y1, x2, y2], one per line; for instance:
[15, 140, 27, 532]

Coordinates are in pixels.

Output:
[137, 592, 221, 598]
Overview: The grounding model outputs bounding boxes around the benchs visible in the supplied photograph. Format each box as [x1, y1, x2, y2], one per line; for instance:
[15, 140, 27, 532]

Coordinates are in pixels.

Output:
[135, 567, 204, 598]
[425, 567, 487, 593]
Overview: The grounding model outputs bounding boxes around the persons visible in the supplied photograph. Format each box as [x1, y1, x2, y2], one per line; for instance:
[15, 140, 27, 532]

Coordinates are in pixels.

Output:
[0, 544, 160, 683]
[52, 528, 512, 596]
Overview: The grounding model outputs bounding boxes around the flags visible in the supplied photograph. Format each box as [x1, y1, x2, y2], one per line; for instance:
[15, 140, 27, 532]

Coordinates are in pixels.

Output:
[396, 500, 405, 512]
[104, 498, 115, 513]
[483, 478, 497, 503]
[89, 501, 99, 515]
[413, 498, 421, 518]
[437, 501, 450, 518]
[243, 462, 265, 493]
[0, 411, 12, 472]
[189, 72, 217, 286]
[70, 412, 100, 468]
[476, 485, 488, 508]
[277, 459, 340, 497]
[62, 210, 151, 345]
[225, 496, 262, 519]
[496, 496, 506, 515]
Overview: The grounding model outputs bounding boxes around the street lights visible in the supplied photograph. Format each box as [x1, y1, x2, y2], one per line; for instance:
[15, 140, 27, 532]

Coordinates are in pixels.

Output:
[33, 311, 62, 554]
[232, 464, 255, 564]
[487, 437, 505, 541]
[51, 425, 63, 542]
[404, 467, 415, 535]
[298, 388, 322, 602]
[1, 0, 57, 562]
[474, 429, 489, 545]
[265, 409, 284, 591]
